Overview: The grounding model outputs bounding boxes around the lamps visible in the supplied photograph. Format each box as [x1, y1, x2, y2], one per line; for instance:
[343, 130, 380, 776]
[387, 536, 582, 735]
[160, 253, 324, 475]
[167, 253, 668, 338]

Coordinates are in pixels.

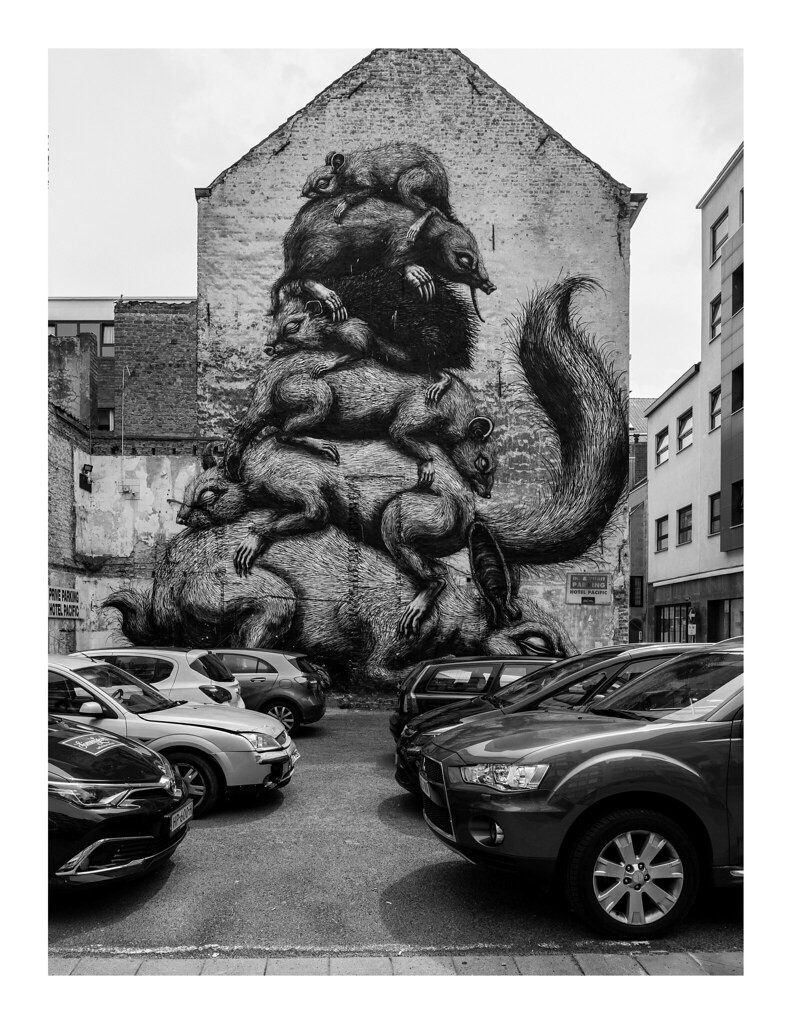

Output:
[83, 463, 94, 473]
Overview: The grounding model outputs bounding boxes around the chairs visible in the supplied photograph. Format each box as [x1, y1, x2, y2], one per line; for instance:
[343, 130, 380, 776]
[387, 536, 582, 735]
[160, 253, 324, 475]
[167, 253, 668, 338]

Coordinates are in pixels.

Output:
[114, 660, 126, 683]
[470, 668, 485, 686]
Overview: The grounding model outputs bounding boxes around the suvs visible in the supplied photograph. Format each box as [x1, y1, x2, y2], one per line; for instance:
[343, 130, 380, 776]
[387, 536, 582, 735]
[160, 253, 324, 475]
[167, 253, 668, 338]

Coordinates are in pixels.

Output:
[196, 649, 327, 735]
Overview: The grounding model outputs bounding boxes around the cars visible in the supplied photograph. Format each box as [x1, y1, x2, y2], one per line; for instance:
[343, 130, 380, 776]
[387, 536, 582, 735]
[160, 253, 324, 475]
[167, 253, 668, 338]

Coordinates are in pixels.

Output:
[389, 636, 743, 940]
[48, 714, 194, 882]
[48, 648, 301, 818]
